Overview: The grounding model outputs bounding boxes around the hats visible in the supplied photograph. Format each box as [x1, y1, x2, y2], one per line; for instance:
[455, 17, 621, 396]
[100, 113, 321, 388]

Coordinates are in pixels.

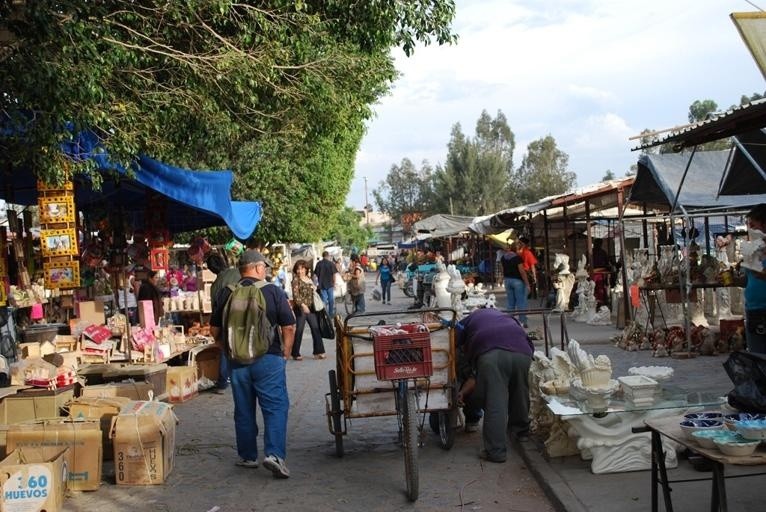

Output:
[239, 250, 274, 268]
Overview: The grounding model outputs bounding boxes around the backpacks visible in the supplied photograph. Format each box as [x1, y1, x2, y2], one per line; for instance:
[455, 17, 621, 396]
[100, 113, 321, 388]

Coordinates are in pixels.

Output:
[222, 279, 277, 366]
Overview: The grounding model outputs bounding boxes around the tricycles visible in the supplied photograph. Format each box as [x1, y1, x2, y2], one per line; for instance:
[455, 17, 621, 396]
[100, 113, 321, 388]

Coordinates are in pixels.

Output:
[322, 307, 462, 502]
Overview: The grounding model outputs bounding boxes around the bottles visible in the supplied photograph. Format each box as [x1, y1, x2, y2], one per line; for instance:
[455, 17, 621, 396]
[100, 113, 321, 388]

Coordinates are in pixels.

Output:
[388, 321, 402, 331]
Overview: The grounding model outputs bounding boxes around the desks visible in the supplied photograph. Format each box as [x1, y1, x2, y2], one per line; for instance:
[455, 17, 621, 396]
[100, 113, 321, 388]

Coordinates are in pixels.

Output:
[630, 410, 766, 511]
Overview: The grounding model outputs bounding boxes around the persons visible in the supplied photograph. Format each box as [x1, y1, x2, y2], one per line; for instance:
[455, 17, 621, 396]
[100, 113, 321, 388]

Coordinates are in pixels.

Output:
[204, 238, 542, 330]
[424, 343, 482, 432]
[203, 253, 242, 391]
[135, 271, 164, 324]
[454, 305, 536, 463]
[587, 238, 608, 268]
[208, 250, 296, 477]
[718, 203, 766, 356]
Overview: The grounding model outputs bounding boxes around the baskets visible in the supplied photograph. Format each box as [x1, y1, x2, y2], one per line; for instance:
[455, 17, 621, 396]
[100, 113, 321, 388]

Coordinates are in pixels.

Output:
[374, 322, 434, 381]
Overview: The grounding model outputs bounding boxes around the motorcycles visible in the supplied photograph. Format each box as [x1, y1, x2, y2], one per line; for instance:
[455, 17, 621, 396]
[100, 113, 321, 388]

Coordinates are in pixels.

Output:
[402, 279, 415, 297]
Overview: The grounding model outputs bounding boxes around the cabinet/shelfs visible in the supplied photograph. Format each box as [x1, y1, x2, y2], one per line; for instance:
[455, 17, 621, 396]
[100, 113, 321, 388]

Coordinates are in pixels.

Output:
[628, 213, 745, 355]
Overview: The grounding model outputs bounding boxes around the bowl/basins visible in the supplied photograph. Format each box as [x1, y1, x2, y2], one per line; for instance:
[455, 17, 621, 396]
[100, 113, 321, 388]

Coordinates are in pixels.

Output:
[681, 409, 766, 458]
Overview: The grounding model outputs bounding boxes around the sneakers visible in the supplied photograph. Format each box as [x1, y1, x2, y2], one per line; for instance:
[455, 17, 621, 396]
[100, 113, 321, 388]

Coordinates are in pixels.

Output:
[464, 421, 481, 432]
[234, 456, 260, 468]
[480, 447, 505, 462]
[263, 454, 291, 478]
[518, 427, 529, 442]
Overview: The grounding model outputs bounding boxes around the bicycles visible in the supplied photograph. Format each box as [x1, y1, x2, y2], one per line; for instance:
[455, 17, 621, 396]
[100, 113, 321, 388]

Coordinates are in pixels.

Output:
[343, 290, 355, 315]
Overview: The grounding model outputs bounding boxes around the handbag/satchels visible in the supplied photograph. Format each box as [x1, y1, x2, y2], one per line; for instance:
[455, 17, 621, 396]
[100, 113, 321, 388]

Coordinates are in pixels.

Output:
[315, 308, 335, 339]
[372, 289, 381, 301]
